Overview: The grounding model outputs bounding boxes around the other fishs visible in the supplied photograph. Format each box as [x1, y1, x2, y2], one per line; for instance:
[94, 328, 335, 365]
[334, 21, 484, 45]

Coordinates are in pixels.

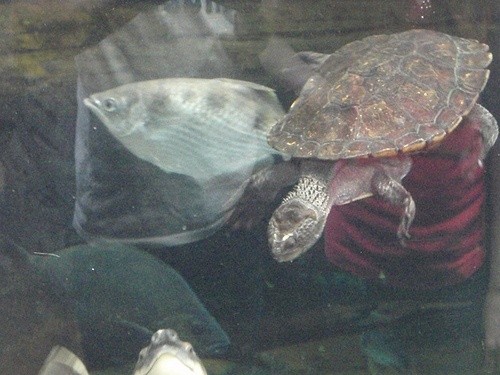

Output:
[132, 329, 208, 375]
[83, 78, 291, 185]
